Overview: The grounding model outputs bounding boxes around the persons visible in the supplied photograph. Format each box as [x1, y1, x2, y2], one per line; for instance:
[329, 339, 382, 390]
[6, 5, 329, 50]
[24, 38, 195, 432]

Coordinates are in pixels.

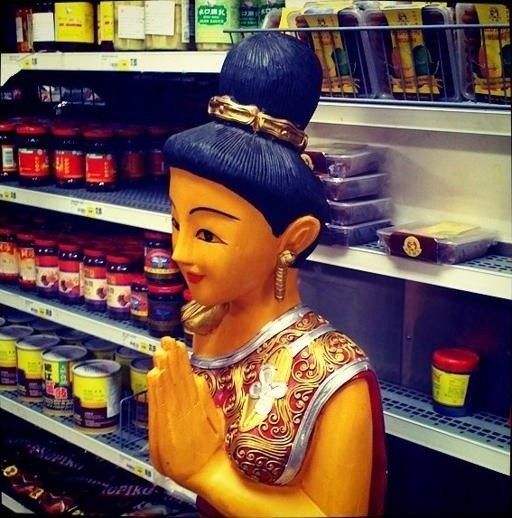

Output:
[147, 32, 389, 518]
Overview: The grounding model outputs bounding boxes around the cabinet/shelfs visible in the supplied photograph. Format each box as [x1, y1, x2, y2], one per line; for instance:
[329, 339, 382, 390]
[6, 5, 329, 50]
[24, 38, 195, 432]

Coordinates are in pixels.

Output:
[224, 26, 512, 475]
[0, 49, 228, 518]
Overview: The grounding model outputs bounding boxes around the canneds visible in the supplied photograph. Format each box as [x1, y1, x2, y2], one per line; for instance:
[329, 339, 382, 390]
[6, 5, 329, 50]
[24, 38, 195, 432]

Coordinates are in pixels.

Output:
[1, 307, 155, 436]
[431, 347, 482, 419]
[0, 223, 189, 342]
[1, 120, 172, 193]
